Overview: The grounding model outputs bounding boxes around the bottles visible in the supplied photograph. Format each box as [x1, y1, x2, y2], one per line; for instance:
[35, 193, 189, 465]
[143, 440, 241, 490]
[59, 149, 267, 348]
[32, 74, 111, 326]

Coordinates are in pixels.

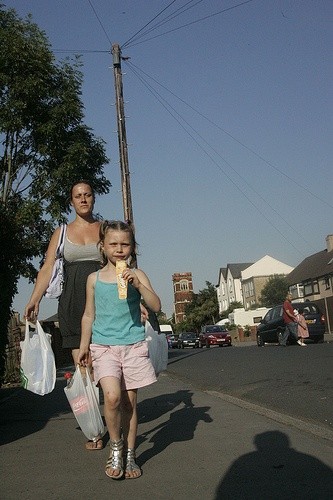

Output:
[65, 372, 101, 440]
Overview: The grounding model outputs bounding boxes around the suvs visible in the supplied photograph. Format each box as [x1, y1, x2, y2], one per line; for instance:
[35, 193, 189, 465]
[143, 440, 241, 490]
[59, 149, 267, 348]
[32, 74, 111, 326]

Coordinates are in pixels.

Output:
[199, 325, 232, 349]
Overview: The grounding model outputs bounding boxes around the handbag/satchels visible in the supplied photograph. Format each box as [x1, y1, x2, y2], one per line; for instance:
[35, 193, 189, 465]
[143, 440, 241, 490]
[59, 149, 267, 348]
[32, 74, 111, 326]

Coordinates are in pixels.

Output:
[20, 318, 56, 395]
[144, 320, 168, 377]
[64, 364, 104, 442]
[44, 223, 66, 299]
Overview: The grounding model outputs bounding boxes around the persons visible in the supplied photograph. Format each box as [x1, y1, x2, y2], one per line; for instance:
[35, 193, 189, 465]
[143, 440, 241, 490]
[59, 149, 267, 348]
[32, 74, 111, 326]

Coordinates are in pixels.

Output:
[165, 334, 171, 349]
[279, 293, 299, 346]
[76, 219, 161, 479]
[24, 181, 108, 450]
[296, 307, 309, 347]
[173, 335, 178, 348]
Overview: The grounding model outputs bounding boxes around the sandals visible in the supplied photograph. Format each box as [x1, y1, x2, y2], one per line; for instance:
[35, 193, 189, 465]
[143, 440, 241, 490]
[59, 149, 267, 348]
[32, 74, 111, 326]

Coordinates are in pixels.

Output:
[104, 439, 124, 479]
[123, 447, 141, 478]
[86, 438, 104, 450]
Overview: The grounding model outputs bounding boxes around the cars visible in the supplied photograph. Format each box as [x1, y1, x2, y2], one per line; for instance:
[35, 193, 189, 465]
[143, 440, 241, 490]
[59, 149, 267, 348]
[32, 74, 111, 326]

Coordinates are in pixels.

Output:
[177, 332, 200, 349]
[167, 334, 179, 349]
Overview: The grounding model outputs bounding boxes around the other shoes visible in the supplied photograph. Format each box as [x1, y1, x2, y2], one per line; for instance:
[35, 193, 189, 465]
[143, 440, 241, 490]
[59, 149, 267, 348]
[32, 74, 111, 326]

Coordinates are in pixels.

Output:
[279, 343, 288, 347]
[301, 343, 307, 347]
[296, 340, 302, 345]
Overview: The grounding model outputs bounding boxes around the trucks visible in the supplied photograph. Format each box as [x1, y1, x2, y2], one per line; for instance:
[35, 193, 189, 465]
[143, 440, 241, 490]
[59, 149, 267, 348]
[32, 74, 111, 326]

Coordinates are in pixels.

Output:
[159, 324, 174, 336]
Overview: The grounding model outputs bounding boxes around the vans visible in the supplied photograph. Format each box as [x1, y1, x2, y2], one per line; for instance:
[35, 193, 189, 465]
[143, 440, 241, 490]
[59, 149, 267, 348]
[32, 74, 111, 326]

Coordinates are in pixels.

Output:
[256, 301, 325, 346]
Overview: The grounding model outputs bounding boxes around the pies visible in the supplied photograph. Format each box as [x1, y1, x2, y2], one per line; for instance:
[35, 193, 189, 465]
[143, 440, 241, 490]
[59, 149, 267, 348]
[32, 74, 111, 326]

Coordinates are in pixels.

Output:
[116, 258, 129, 300]
